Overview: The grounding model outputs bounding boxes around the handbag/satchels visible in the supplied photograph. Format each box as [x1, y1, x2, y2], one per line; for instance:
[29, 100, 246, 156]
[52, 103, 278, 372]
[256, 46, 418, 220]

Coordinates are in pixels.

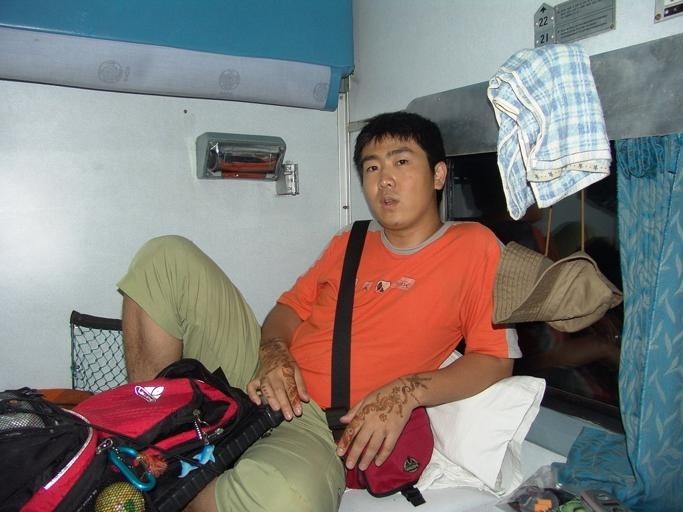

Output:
[326, 406, 434, 497]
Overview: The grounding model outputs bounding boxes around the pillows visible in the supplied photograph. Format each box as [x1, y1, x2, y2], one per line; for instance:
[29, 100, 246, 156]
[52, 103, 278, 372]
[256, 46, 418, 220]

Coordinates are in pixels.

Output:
[409, 349, 548, 491]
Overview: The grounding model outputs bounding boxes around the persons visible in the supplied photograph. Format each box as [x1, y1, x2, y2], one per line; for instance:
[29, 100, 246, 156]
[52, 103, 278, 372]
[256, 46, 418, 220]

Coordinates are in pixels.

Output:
[455, 154, 622, 415]
[115, 110, 524, 511]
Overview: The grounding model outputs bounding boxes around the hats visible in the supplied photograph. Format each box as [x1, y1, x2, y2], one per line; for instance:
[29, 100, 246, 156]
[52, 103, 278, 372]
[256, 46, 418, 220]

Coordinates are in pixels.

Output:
[492, 241, 624, 333]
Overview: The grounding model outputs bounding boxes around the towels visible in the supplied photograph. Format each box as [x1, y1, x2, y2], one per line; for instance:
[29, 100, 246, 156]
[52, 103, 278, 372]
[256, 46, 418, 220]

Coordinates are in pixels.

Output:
[487, 41, 614, 222]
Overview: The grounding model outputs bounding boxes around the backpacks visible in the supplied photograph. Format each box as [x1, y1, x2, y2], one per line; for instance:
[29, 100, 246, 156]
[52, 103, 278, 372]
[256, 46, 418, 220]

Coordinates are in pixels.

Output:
[0, 358, 266, 512]
